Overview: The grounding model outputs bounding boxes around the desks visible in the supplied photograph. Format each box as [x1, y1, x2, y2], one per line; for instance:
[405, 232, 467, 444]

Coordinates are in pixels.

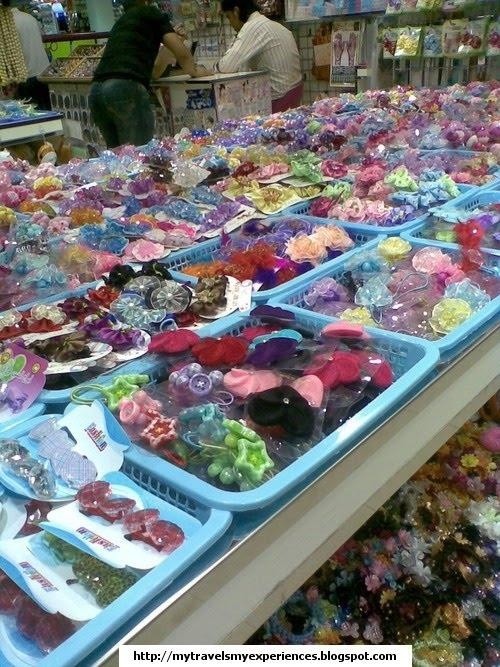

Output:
[0, 110, 64, 148]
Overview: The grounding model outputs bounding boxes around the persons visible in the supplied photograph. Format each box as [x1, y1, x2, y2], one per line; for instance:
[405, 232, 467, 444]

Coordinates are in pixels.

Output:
[3, 3, 50, 108]
[215, 0, 302, 111]
[89, 0, 210, 146]
